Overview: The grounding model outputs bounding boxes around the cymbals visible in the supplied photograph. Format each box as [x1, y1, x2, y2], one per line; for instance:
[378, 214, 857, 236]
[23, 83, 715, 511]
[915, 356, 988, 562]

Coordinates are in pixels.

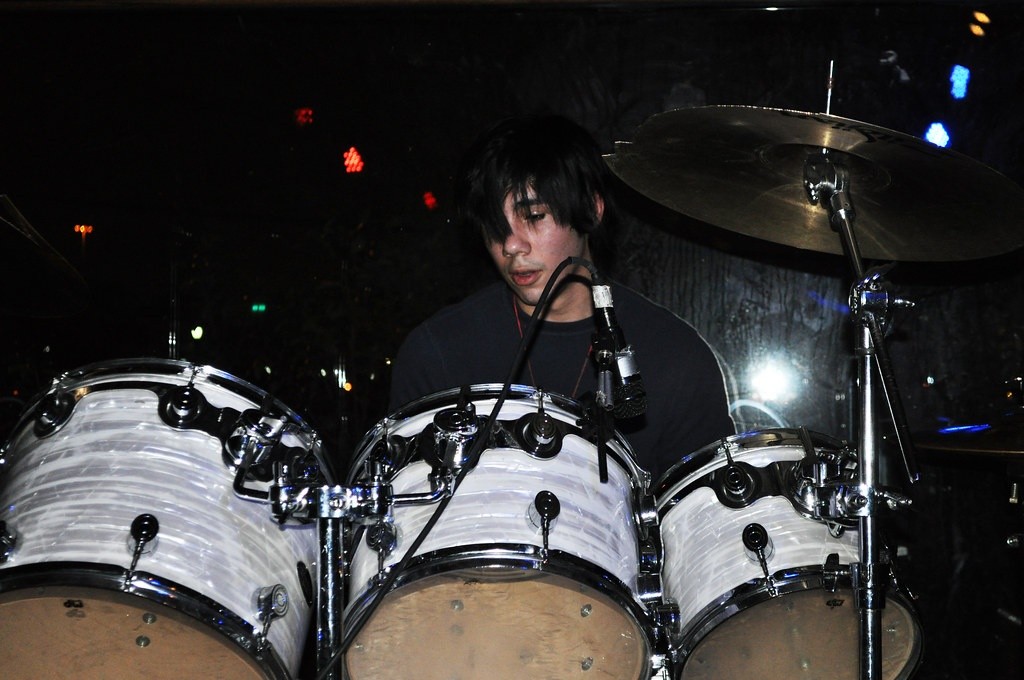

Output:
[887, 417, 1024, 455]
[604, 100, 1024, 268]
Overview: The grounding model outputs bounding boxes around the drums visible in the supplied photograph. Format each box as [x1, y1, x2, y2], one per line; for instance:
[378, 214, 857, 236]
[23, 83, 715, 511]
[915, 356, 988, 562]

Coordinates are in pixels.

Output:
[658, 424, 923, 679]
[2, 357, 337, 680]
[341, 380, 660, 680]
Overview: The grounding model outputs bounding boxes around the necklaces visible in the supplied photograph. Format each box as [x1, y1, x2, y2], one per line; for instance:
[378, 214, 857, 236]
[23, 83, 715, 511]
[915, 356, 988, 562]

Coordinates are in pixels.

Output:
[513, 295, 593, 410]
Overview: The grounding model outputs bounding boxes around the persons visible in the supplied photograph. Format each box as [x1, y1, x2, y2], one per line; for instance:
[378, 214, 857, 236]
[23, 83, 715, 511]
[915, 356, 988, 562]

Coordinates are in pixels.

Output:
[389, 118, 739, 491]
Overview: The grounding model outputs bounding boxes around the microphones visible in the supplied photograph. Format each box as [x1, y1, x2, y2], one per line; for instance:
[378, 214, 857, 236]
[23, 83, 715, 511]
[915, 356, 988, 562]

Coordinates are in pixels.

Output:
[592, 270, 649, 418]
